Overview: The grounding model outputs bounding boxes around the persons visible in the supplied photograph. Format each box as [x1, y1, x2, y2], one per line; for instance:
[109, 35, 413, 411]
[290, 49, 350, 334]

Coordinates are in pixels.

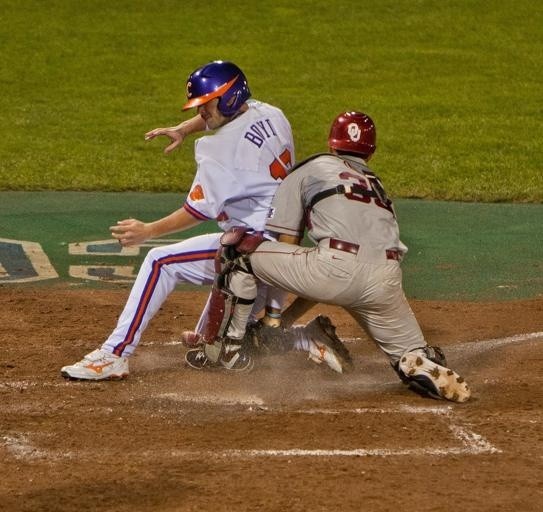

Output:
[186, 110, 472, 402]
[60, 60, 355, 381]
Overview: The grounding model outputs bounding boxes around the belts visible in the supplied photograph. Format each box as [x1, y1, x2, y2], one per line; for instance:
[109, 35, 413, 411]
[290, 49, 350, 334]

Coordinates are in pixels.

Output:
[330, 238, 398, 261]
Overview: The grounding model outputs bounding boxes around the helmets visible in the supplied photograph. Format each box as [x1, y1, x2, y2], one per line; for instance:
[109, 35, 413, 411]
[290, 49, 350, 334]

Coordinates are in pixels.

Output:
[329, 111, 376, 156]
[182, 61, 251, 117]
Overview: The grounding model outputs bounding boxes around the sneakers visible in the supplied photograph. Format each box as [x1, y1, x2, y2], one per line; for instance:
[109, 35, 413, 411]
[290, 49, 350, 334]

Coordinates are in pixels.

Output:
[180, 315, 355, 374]
[399, 353, 471, 403]
[60, 348, 130, 381]
